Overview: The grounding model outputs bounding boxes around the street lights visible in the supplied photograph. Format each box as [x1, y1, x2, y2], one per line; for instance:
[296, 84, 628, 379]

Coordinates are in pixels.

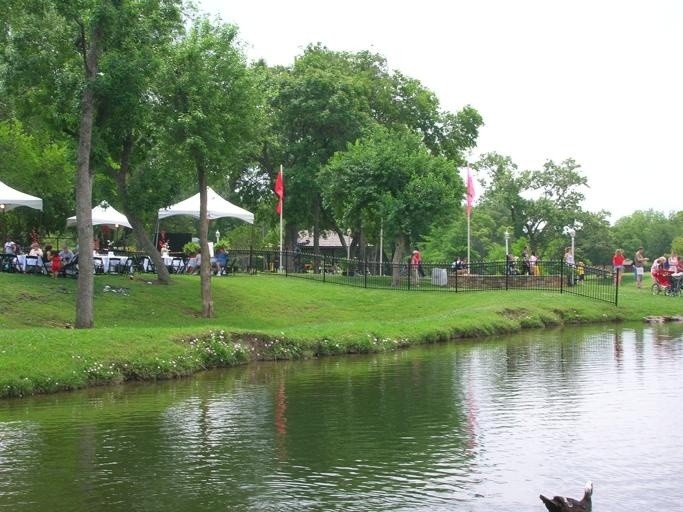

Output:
[570, 227, 576, 285]
[505, 230, 510, 255]
[215, 229, 220, 243]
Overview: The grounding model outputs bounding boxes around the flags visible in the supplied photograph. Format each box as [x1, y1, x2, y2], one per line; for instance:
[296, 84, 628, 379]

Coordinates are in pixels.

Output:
[466, 167, 476, 215]
[275, 172, 283, 215]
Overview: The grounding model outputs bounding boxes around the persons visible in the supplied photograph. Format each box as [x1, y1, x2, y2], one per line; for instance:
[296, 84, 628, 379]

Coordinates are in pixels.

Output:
[612, 248, 625, 287]
[192, 248, 202, 275]
[220, 247, 230, 275]
[415, 246, 427, 277]
[411, 251, 419, 287]
[94, 233, 100, 254]
[161, 242, 180, 273]
[452, 256, 468, 274]
[651, 250, 683, 296]
[633, 246, 649, 288]
[215, 249, 227, 276]
[506, 246, 540, 276]
[577, 262, 585, 285]
[4, 237, 80, 279]
[562, 246, 576, 285]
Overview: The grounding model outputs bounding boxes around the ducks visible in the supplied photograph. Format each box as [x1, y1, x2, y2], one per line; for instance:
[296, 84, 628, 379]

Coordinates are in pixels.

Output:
[539, 482, 595, 512]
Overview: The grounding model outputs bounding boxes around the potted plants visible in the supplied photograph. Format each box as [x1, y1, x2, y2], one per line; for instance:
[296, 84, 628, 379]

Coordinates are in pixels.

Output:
[338, 257, 355, 276]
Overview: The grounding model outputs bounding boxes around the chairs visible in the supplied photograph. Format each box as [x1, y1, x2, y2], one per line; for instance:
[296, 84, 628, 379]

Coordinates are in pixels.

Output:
[0, 252, 237, 276]
